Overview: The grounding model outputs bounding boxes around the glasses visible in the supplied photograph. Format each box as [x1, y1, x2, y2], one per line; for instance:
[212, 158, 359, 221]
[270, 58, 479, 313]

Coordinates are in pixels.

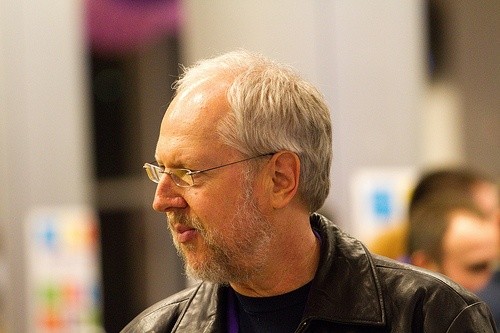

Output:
[142, 152, 273, 188]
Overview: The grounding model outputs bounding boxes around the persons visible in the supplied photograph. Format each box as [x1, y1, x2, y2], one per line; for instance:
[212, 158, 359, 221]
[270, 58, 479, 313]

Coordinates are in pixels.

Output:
[403, 168, 500, 333]
[118, 47, 500, 333]
